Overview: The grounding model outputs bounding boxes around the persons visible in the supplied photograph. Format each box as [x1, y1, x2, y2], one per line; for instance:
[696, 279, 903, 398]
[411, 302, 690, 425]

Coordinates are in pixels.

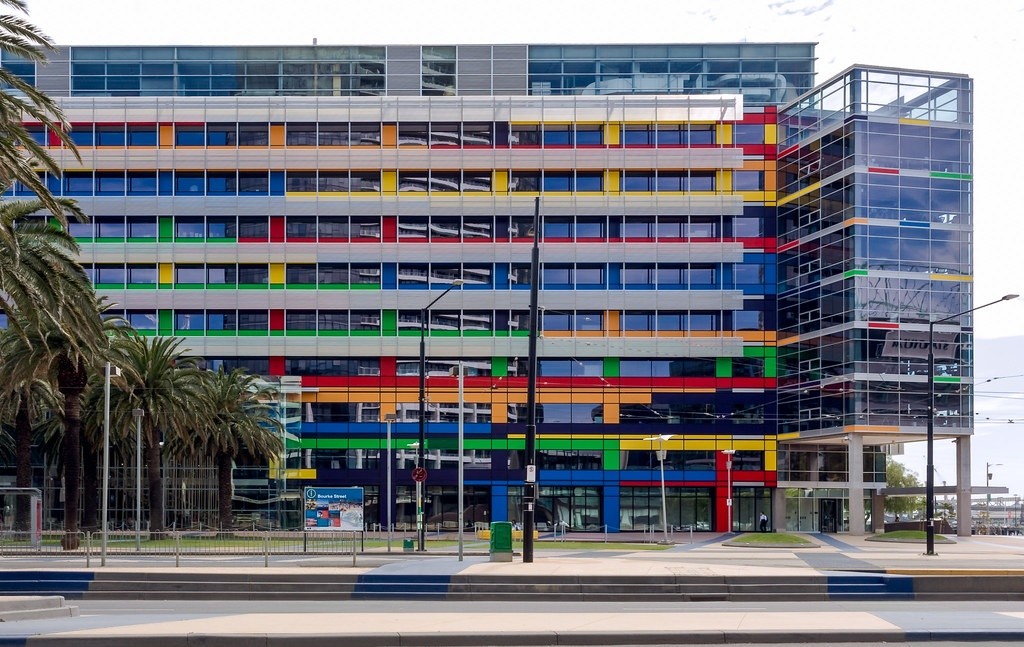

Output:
[759, 512, 768, 532]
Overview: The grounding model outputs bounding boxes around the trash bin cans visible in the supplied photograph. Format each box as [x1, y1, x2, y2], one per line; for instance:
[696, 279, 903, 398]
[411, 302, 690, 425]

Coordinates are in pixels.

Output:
[488, 521, 514, 561]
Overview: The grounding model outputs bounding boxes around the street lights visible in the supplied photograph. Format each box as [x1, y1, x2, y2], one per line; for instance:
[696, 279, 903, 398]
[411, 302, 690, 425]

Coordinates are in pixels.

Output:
[521, 196, 541, 562]
[416, 278, 464, 552]
[986, 461, 1004, 535]
[925, 293, 1022, 556]
